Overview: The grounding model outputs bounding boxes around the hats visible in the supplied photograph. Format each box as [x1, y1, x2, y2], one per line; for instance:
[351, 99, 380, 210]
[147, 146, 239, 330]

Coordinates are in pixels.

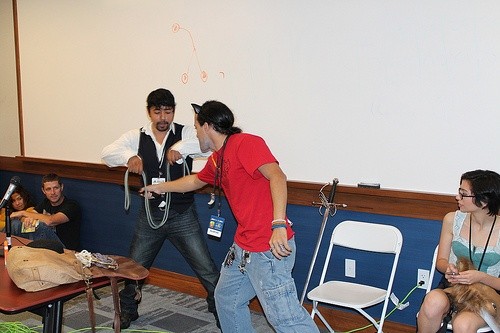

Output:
[191, 99, 235, 130]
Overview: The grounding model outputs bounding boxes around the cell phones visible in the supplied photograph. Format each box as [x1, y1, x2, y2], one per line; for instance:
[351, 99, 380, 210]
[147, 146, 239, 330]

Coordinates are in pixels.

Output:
[444, 272, 458, 275]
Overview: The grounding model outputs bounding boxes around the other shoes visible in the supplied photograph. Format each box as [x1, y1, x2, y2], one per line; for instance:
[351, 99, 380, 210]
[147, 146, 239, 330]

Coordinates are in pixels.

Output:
[113, 308, 139, 329]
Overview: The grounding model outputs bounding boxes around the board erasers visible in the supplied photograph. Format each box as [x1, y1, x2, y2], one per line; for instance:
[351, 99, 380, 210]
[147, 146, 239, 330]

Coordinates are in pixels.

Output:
[357, 182, 381, 187]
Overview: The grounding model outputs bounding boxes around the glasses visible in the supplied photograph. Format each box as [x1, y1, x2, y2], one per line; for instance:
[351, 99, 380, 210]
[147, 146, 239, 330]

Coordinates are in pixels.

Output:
[458, 192, 477, 200]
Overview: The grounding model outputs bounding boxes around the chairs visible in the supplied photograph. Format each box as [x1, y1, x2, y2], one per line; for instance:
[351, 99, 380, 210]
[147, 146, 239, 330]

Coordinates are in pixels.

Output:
[307, 221, 403, 333]
[416, 244, 492, 333]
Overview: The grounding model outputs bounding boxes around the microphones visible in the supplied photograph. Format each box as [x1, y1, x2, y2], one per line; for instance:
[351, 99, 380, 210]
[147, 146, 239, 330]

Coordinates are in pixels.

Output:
[0, 176, 22, 211]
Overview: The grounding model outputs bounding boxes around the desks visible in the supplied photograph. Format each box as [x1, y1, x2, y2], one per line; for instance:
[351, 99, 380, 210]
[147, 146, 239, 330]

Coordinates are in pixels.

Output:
[0, 232, 134, 333]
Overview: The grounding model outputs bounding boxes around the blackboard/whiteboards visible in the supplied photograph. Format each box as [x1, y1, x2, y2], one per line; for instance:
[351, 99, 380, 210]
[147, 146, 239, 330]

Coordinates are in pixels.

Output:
[12, 0, 500, 203]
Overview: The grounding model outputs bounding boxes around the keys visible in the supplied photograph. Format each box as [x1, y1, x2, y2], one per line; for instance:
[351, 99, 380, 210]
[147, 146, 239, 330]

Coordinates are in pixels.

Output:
[224, 254, 235, 267]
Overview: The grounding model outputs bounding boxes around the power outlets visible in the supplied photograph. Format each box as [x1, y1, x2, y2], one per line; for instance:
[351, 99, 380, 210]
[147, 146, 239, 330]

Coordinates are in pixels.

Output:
[345, 259, 356, 278]
[417, 269, 430, 289]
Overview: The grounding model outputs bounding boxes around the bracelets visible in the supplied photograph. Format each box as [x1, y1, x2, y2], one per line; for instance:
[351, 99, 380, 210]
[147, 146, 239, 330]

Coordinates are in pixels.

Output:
[271, 219, 287, 230]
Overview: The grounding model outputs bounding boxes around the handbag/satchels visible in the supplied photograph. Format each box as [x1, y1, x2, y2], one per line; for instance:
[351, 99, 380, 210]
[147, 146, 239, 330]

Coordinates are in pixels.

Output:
[6, 244, 150, 293]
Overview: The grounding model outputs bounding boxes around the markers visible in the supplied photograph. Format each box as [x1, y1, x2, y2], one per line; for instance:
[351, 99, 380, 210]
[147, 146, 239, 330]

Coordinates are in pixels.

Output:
[3, 241, 8, 266]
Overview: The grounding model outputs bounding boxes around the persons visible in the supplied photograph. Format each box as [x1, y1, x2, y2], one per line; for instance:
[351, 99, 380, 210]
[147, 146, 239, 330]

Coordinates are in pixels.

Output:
[137, 100, 320, 333]
[0, 185, 42, 231]
[417, 170, 500, 333]
[9, 174, 82, 253]
[100, 89, 223, 333]
